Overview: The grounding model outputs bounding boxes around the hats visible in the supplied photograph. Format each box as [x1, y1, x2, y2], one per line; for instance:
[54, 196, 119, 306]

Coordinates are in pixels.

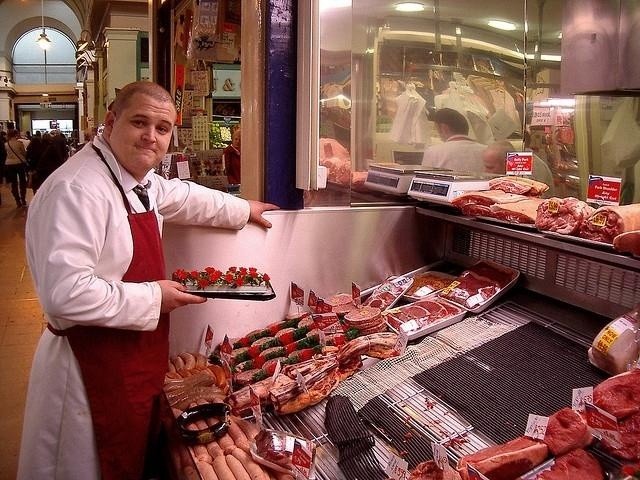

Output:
[427, 108, 469, 134]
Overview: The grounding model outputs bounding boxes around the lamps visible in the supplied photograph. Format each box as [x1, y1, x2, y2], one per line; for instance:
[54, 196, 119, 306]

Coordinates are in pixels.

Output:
[75, 28, 106, 73]
[35, 0, 52, 50]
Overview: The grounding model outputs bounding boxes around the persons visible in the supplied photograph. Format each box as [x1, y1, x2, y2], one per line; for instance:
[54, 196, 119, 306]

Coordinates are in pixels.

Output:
[421, 107, 558, 200]
[222, 129, 240, 185]
[0, 126, 69, 208]
[16, 80, 282, 480]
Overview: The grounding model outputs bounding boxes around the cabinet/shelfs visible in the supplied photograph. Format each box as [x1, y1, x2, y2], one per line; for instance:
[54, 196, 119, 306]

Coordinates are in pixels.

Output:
[156, 256, 640, 480]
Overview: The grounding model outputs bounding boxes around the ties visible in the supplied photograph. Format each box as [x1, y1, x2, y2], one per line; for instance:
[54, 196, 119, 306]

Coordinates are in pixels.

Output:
[131, 179, 152, 212]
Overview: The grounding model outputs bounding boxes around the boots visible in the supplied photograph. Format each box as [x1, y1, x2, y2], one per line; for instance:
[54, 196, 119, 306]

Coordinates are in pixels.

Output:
[11, 189, 27, 209]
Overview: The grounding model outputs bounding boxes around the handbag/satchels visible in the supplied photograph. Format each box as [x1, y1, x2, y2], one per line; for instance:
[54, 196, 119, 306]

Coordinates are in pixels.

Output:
[23, 158, 32, 173]
[25, 170, 42, 188]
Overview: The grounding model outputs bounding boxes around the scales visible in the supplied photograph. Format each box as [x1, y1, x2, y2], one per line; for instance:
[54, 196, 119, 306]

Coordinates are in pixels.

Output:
[407, 173, 506, 207]
[364, 164, 453, 197]
